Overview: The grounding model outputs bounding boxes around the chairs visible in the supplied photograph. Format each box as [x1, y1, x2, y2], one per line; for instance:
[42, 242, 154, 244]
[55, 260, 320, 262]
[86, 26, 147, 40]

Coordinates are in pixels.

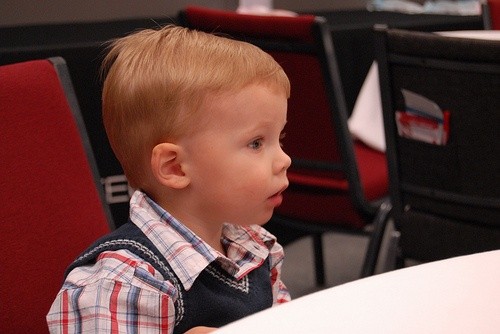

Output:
[0, 56, 116, 334]
[175, 6, 500, 286]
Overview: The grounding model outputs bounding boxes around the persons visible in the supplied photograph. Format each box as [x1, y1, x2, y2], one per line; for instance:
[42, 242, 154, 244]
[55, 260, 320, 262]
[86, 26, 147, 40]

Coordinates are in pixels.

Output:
[46, 24, 292, 334]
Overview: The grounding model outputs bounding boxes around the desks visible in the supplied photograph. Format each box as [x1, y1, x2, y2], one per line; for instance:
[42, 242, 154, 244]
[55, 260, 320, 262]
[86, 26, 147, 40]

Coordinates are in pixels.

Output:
[0, 0, 484, 247]
[208, 249, 500, 334]
